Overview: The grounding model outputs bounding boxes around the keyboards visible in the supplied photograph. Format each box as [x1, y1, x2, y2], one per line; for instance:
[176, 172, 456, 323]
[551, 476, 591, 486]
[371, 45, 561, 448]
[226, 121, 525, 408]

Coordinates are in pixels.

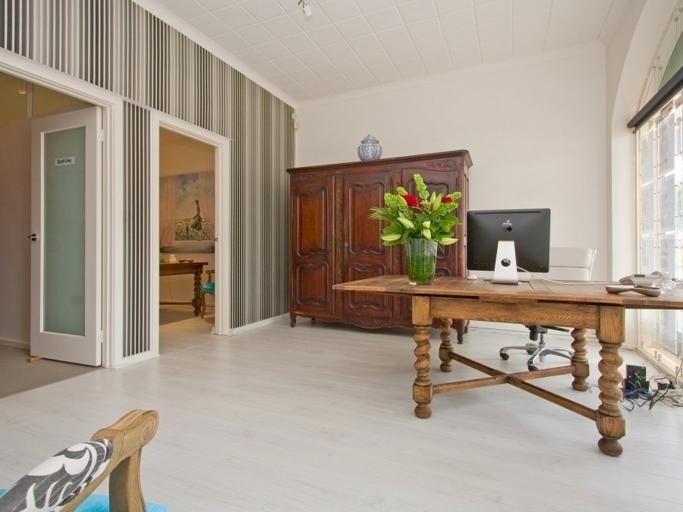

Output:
[482, 275, 531, 282]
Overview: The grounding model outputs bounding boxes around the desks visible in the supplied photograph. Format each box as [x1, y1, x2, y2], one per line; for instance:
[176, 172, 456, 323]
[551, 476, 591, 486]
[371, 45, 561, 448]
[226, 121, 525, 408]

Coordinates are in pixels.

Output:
[159, 260, 208, 318]
[332, 274, 683, 457]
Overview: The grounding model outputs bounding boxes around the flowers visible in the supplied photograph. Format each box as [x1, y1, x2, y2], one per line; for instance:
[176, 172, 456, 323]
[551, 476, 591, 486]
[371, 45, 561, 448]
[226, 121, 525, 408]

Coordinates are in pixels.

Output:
[367, 172, 465, 278]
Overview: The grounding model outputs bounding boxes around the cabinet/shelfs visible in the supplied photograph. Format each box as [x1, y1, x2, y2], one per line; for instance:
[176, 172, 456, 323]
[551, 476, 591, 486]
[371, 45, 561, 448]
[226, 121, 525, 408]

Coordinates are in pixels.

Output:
[286, 150, 474, 334]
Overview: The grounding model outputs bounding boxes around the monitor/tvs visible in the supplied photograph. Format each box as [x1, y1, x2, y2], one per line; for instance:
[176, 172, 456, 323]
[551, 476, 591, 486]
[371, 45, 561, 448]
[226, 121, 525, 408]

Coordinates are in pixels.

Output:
[467, 208, 550, 284]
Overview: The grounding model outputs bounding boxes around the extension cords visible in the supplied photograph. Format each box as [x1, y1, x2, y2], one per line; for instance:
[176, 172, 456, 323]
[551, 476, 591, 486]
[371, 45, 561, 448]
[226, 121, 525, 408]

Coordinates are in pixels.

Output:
[658, 387, 683, 397]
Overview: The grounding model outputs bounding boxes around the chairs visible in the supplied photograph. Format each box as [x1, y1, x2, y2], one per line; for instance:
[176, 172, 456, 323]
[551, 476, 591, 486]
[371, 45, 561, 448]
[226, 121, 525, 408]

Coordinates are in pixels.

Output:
[198, 270, 214, 318]
[0, 407, 166, 512]
[499, 245, 597, 372]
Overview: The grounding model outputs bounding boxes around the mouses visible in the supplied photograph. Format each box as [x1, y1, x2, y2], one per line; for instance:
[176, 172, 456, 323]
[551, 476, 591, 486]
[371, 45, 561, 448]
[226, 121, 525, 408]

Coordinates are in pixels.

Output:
[467, 274, 477, 280]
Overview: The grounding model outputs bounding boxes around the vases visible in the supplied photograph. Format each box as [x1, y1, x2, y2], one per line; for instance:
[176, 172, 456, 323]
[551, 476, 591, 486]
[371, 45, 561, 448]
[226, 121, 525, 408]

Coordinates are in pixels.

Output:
[403, 239, 438, 285]
